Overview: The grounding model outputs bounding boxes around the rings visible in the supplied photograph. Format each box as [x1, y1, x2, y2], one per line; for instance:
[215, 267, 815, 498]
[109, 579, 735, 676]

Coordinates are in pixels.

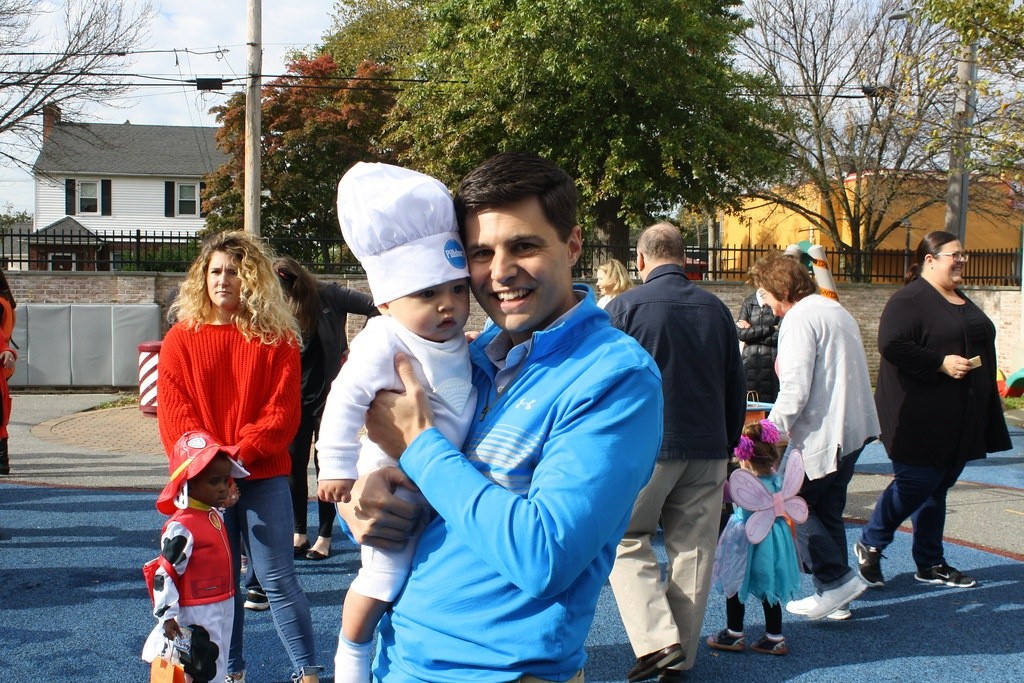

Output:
[9, 358, 13, 361]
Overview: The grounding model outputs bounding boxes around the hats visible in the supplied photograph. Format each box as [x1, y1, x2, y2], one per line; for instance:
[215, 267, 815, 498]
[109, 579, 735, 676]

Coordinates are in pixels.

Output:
[335, 161, 474, 307]
[783, 244, 802, 261]
[156, 431, 250, 515]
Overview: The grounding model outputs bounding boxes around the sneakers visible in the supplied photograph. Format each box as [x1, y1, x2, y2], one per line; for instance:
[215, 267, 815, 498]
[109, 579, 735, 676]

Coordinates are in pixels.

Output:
[807, 575, 869, 621]
[786, 596, 851, 620]
[915, 562, 977, 587]
[853, 540, 886, 586]
[706, 628, 746, 650]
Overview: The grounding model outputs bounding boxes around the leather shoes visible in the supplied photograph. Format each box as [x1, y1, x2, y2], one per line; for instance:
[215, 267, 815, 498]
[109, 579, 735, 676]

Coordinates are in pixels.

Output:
[628, 644, 686, 683]
[658, 668, 689, 683]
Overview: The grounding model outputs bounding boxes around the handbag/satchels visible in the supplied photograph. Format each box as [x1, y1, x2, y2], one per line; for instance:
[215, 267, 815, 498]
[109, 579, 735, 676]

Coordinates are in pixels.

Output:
[151, 634, 185, 683]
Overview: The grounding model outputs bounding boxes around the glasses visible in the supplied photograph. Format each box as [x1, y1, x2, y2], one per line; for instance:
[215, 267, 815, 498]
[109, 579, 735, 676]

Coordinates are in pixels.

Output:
[935, 250, 971, 264]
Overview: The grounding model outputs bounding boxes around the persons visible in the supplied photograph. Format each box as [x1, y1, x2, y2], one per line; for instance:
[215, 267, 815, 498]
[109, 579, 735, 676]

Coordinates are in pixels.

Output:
[853, 231, 1012, 587]
[334, 150, 664, 683]
[0, 267, 16, 474]
[241, 246, 883, 654]
[0, 329, 18, 367]
[314, 161, 478, 683]
[157, 229, 320, 683]
[603, 221, 746, 683]
[153, 431, 239, 683]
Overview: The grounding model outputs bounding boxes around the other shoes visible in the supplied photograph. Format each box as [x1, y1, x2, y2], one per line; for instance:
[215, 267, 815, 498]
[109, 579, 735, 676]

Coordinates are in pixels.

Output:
[750, 633, 788, 654]
[304, 540, 332, 560]
[292, 537, 312, 561]
[225, 670, 246, 683]
[244, 586, 268, 610]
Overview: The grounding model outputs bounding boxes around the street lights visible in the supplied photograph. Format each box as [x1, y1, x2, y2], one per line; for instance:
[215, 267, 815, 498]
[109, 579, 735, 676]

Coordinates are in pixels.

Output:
[747, 217, 752, 275]
[897, 218, 912, 282]
[889, 6, 980, 250]
